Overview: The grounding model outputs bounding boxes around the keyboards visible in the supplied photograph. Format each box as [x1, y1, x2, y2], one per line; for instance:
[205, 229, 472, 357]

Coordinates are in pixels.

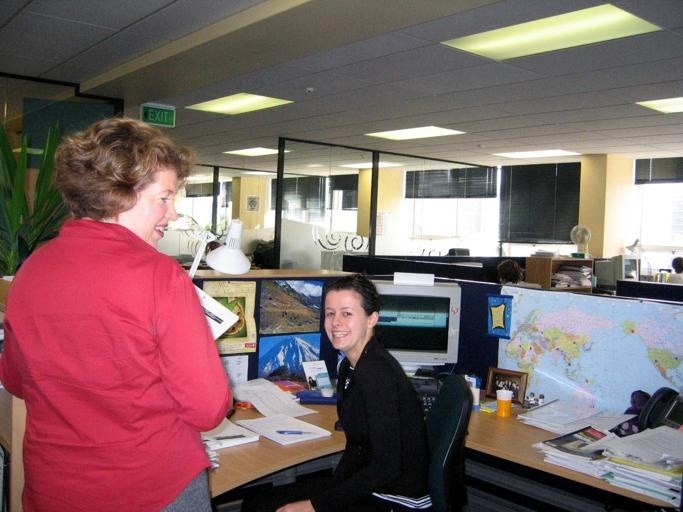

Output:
[408, 379, 441, 415]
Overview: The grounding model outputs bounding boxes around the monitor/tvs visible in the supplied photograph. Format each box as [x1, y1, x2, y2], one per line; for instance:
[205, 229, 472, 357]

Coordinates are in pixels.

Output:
[373, 282, 462, 380]
[449, 248, 469, 257]
[595, 255, 639, 286]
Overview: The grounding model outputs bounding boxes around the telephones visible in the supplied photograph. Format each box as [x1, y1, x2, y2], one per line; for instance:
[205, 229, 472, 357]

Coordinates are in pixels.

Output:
[638, 387, 683, 431]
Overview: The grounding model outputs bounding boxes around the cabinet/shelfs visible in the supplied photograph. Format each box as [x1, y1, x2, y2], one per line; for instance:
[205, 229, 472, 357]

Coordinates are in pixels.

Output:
[524, 255, 594, 293]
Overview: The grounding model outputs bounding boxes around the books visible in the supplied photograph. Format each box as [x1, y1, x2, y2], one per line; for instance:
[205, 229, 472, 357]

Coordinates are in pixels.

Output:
[530, 423, 681, 508]
[301, 360, 332, 390]
[551, 264, 592, 288]
[200, 416, 259, 451]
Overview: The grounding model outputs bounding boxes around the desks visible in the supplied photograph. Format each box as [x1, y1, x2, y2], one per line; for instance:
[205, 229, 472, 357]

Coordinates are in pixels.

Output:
[202, 397, 682, 511]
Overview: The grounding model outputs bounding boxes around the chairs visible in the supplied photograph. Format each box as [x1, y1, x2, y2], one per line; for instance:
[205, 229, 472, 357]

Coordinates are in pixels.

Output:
[425, 370, 474, 511]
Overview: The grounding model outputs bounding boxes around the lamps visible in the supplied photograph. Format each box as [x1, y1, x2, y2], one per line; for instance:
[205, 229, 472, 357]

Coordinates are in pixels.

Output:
[187, 217, 252, 281]
[625, 238, 652, 276]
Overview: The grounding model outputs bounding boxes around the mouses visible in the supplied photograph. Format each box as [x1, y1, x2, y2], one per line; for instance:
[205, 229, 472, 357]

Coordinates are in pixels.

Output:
[320, 387, 334, 398]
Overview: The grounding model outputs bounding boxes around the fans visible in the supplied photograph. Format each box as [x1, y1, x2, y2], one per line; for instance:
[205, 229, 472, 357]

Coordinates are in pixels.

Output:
[570, 224, 591, 259]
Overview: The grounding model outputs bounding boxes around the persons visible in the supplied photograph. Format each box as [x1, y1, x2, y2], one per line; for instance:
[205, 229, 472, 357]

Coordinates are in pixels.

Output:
[0, 116, 234, 511]
[308, 376, 317, 391]
[490, 375, 519, 396]
[239, 272, 434, 511]
[666, 255, 682, 286]
[494, 258, 540, 289]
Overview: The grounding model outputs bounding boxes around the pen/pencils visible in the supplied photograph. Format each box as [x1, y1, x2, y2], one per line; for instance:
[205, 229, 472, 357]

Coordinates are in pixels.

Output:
[277, 431, 302, 434]
[216, 435, 245, 440]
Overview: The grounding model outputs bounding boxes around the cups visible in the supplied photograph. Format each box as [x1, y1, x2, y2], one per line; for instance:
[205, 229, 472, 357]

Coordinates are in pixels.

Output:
[496, 390, 513, 418]
